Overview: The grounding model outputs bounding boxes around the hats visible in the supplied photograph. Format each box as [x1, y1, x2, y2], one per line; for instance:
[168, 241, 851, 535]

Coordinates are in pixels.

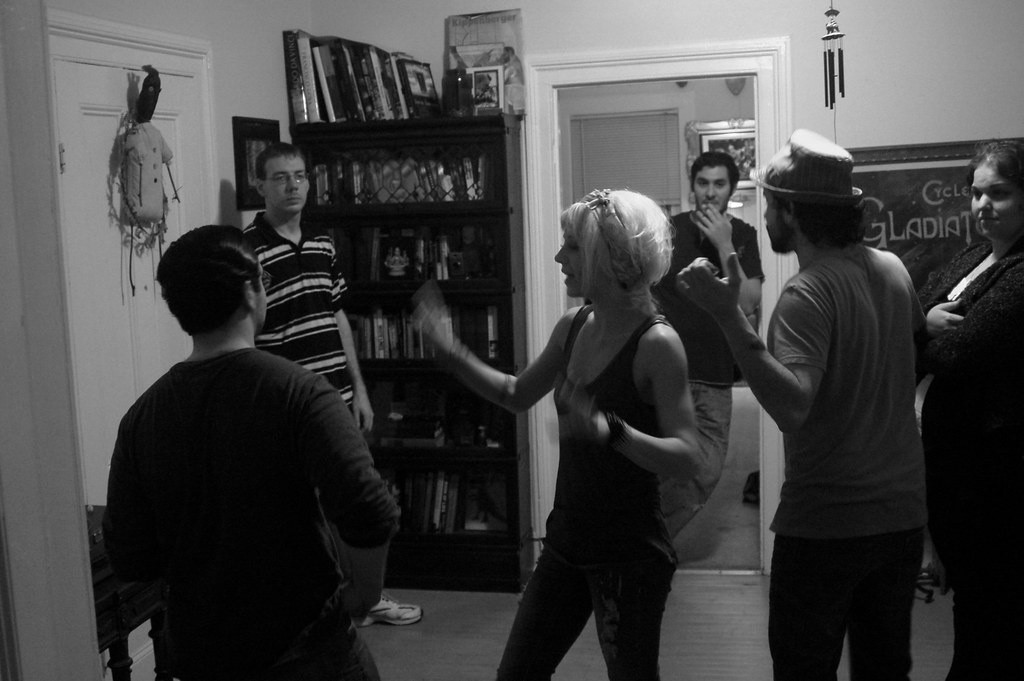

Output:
[749, 129, 863, 206]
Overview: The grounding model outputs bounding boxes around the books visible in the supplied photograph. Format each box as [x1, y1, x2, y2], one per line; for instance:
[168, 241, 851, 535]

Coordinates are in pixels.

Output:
[246, 27, 507, 536]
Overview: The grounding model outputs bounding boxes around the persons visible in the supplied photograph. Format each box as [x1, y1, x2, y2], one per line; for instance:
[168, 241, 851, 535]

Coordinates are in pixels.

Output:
[918, 139, 1024, 681]
[678, 130, 930, 681]
[99, 224, 404, 680]
[414, 182, 701, 681]
[646, 149, 765, 540]
[239, 141, 424, 628]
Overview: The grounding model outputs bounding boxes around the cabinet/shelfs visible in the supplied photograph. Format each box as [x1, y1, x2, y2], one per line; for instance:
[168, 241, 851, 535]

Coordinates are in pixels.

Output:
[294, 114, 534, 594]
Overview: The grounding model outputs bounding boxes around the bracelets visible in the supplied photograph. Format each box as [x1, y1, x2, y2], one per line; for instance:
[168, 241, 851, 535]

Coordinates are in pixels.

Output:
[603, 410, 625, 448]
[731, 337, 765, 372]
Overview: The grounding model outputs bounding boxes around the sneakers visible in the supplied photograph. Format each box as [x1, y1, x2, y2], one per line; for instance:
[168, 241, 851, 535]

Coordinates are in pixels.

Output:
[354, 594, 423, 627]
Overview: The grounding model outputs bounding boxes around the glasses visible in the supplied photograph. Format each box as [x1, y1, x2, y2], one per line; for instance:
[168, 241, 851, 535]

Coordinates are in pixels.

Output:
[252, 270, 271, 291]
[266, 173, 309, 184]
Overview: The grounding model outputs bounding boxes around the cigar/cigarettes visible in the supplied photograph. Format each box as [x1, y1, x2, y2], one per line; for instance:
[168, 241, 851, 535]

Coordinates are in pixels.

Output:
[702, 206, 709, 210]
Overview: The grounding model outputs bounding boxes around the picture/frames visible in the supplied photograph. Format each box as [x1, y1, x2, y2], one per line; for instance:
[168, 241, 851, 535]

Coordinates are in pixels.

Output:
[467, 65, 505, 115]
[233, 115, 281, 211]
[683, 118, 756, 189]
